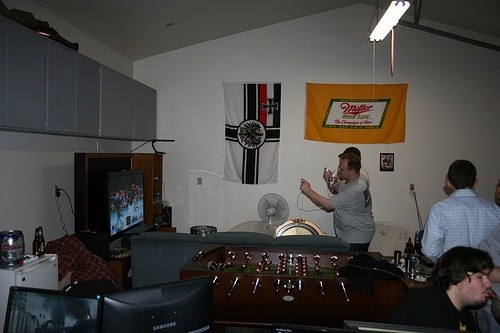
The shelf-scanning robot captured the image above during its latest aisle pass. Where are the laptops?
[3,285,105,333]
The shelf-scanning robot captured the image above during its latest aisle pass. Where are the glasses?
[339,167,354,172]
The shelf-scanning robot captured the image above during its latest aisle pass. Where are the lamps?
[370,0,410,42]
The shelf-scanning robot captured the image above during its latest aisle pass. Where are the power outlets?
[55,186,58,195]
[409,184,415,190]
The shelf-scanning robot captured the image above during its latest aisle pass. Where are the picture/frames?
[380,153,394,172]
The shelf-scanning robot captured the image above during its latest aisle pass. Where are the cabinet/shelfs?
[74,151,176,284]
[0,16,156,142]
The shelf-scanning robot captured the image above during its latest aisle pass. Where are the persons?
[386,246,495,330]
[421,159,500,263]
[113,183,143,225]
[301,147,376,252]
[477,178,500,333]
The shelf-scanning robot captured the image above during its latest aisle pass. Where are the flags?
[305,82,408,144]
[222,81,281,184]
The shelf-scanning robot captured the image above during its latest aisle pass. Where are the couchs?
[128,230,351,288]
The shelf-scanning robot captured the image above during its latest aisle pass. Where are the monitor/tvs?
[101,275,214,333]
[100,168,146,240]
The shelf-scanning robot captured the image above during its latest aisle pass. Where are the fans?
[257,193,289,228]
